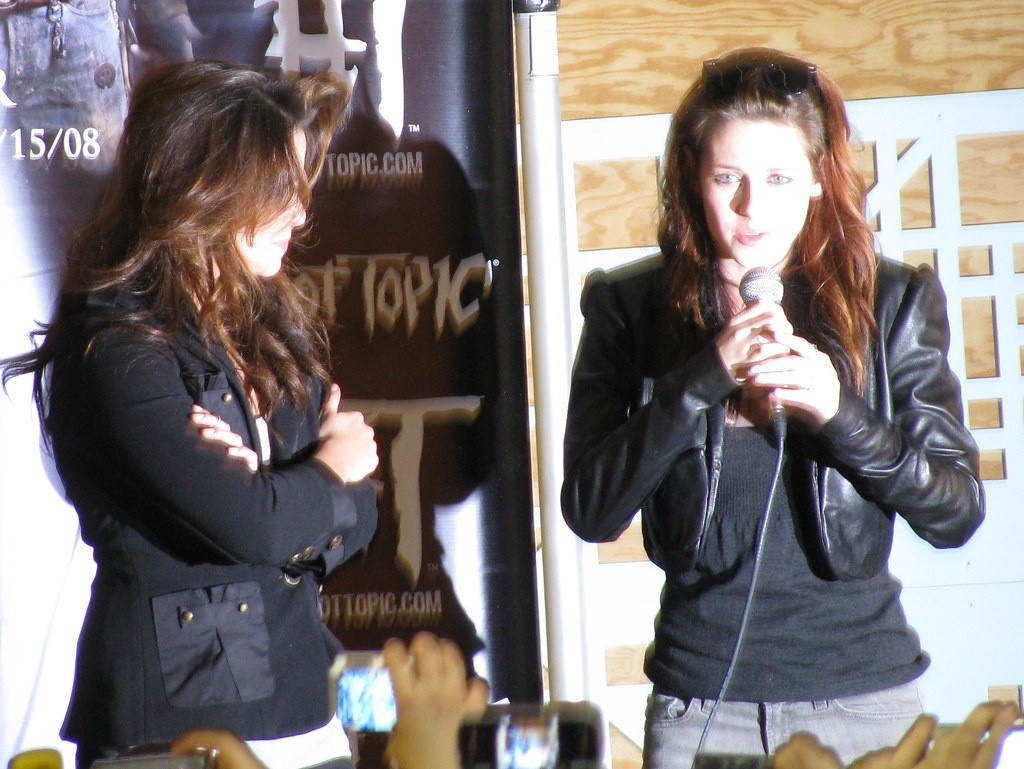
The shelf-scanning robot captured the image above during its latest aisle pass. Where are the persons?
[560,46,987,769]
[0,57,381,769]
[174,632,1020,769]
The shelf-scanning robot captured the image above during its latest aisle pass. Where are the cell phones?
[327,653,399,732]
[451,701,612,769]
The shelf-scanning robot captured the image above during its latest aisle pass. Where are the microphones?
[740,266,786,440]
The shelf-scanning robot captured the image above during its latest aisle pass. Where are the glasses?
[701,59,822,99]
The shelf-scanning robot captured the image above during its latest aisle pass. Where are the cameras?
[91,752,214,769]
